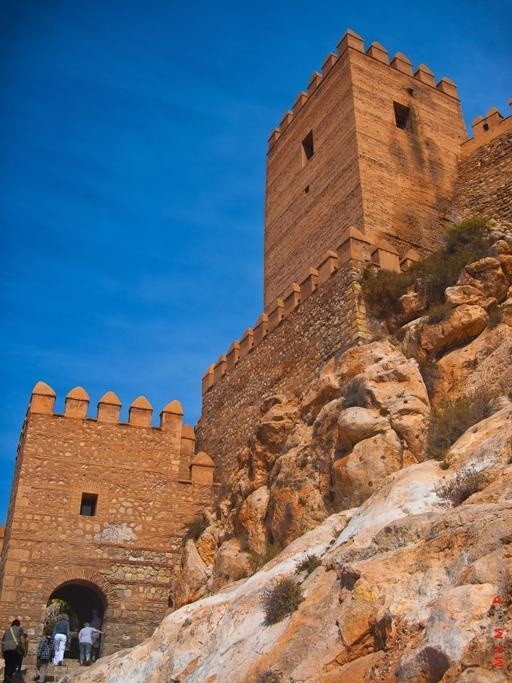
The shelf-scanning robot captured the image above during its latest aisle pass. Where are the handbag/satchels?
[15,642,24,655]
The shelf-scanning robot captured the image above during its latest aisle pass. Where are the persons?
[89,611,101,662]
[1,619,27,682]
[37,627,55,683]
[52,614,70,666]
[78,623,104,666]
